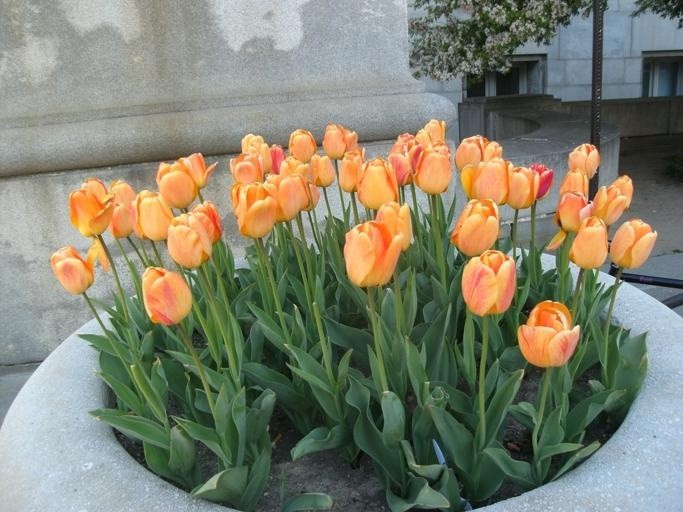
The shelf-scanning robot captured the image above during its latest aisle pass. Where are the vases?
[1,248,683,511]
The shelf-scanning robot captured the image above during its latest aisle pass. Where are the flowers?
[52,118,657,512]
